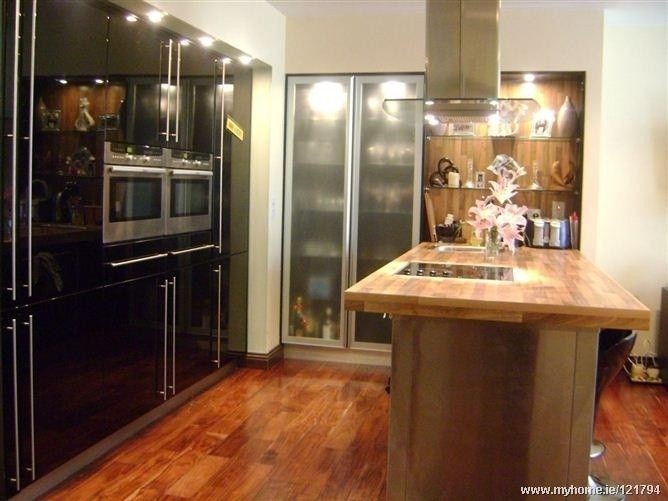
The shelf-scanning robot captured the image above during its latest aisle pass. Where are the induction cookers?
[400,261,515,282]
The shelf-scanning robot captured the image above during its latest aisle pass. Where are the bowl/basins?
[436,224,459,241]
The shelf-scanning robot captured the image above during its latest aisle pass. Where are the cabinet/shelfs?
[213,250,251,376]
[0,287,113,500]
[110,6,216,152]
[416,73,585,249]
[214,58,254,261]
[0,0,114,311]
[105,263,214,440]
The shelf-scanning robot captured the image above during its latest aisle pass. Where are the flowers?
[459,148,529,253]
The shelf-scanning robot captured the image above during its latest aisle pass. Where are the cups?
[448,172,459,187]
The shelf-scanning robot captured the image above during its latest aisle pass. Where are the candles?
[447,171,459,189]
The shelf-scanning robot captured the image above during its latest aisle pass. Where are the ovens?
[102,139,214,255]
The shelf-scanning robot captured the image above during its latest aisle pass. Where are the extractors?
[382,0,540,130]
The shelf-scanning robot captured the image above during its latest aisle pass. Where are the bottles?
[36,99,50,130]
[289,297,339,339]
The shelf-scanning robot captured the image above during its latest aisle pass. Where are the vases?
[483,231,504,261]
